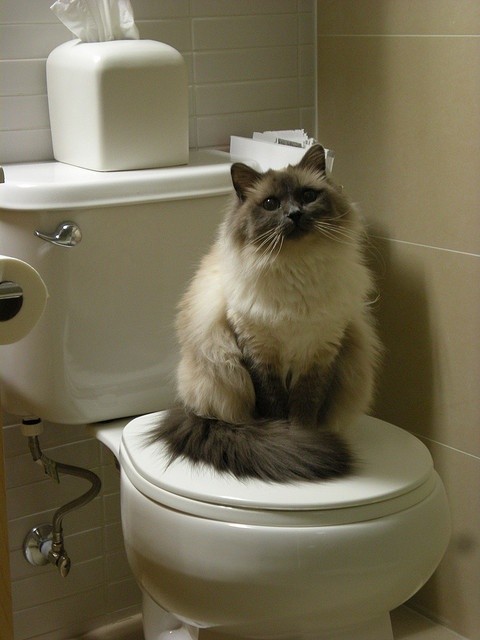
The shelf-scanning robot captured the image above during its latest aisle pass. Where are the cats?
[136,142,385,487]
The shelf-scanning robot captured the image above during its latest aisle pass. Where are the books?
[228,127,335,180]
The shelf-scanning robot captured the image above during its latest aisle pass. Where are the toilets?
[0,149,452,640]
